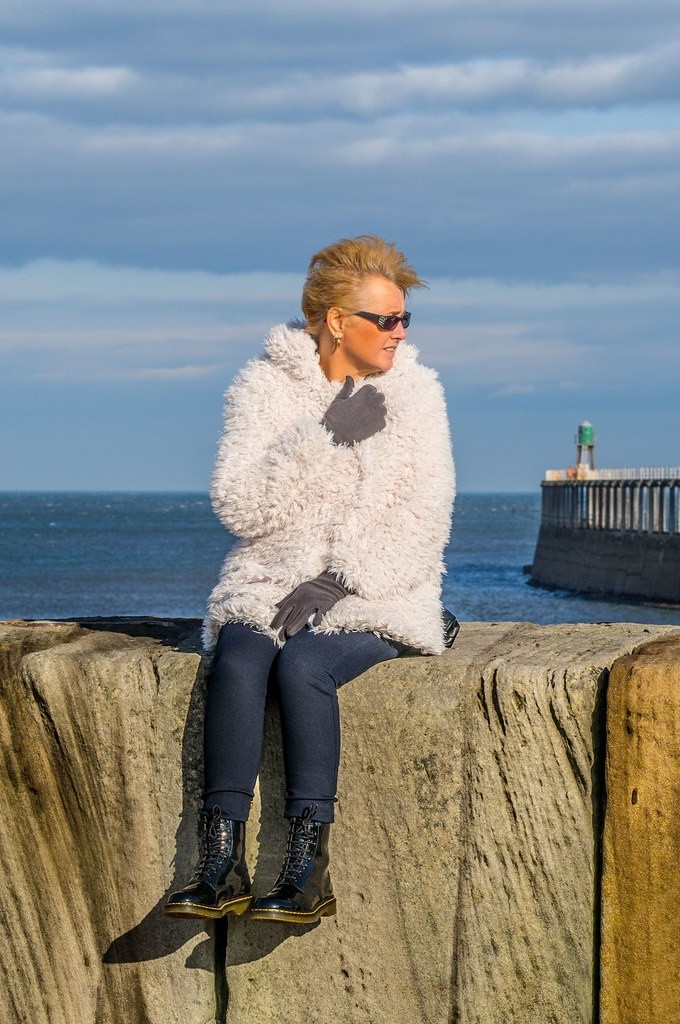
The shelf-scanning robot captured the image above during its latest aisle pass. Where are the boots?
[164,803,337,923]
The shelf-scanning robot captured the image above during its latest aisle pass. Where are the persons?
[162,235,456,926]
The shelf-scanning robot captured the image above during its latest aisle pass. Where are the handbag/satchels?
[441,608,460,648]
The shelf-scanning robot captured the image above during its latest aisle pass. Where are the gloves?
[270,566,356,637]
[319,375,387,448]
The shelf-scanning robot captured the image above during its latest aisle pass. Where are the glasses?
[324,307,411,330]
